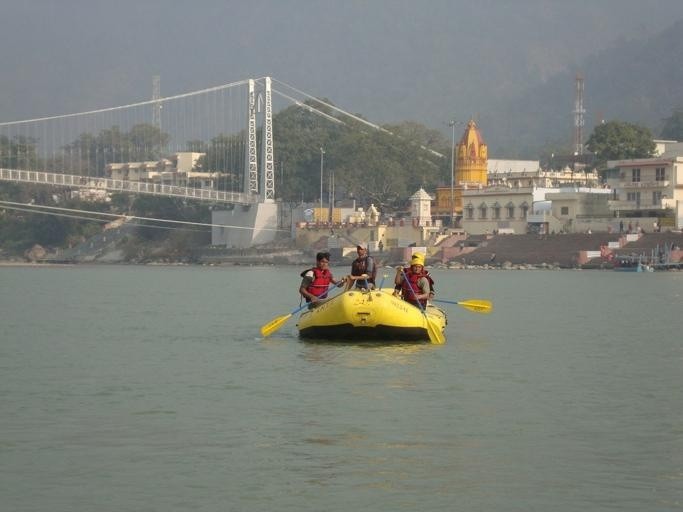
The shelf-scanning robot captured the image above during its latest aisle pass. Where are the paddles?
[401,266,446,345]
[260,278,348,337]
[432,298,491,314]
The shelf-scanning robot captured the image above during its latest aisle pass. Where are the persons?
[299,252,344,309]
[392,252,435,310]
[348,242,377,290]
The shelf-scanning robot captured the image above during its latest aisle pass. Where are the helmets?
[409,252,425,267]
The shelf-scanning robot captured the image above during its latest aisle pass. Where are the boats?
[613,254,648,272]
[296,288,448,342]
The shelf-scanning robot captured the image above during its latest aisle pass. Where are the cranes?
[550,151,598,184]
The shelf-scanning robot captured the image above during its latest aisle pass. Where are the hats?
[355,241,367,251]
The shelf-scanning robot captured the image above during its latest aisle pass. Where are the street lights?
[319,147,326,224]
[448,119,458,228]
